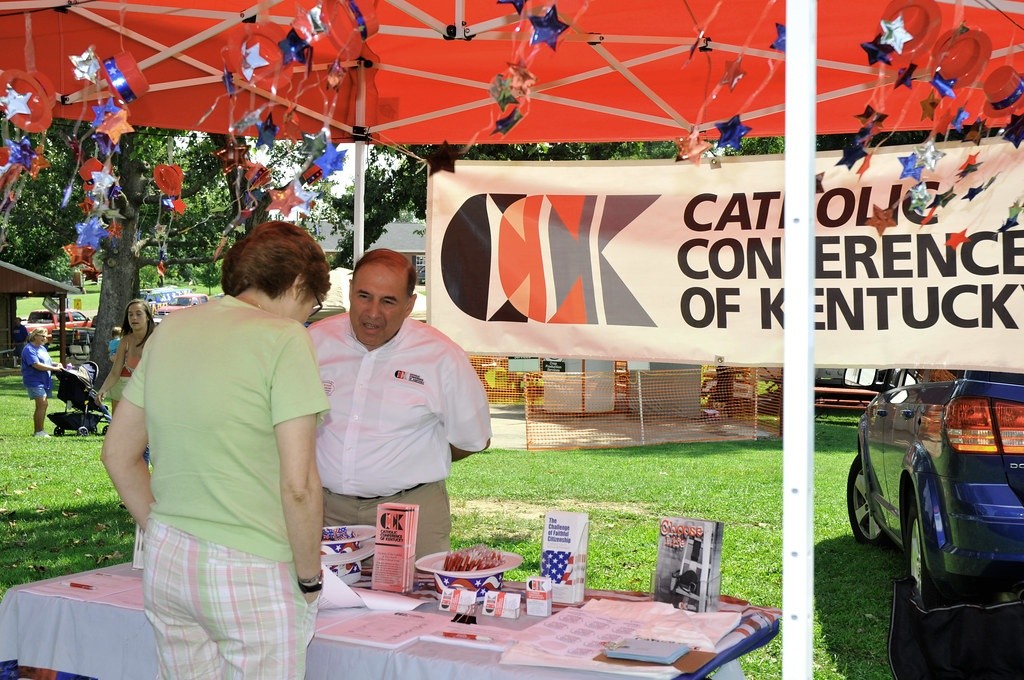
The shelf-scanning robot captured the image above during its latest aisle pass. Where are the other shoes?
[34,431,50,437]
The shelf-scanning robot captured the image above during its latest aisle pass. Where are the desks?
[0,561,782,680]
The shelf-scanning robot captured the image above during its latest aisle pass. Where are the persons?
[100,221,330,680]
[12,318,29,368]
[95,298,156,506]
[21,327,63,437]
[305,248,493,564]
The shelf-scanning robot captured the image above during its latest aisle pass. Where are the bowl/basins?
[414,552,524,602]
[321,525,376,586]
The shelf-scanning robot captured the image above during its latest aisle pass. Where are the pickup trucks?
[50,315,99,356]
[20,310,94,348]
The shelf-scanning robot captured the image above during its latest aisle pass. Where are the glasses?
[310,290,323,316]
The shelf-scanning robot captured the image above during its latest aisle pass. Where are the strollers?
[51,359,115,436]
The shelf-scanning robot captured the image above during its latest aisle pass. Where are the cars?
[156,294,209,318]
[303,304,346,328]
[846,371,1024,601]
[141,287,192,306]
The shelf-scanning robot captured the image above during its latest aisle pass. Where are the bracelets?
[298,570,324,594]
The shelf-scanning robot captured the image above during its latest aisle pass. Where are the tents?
[0,0,1024,680]
